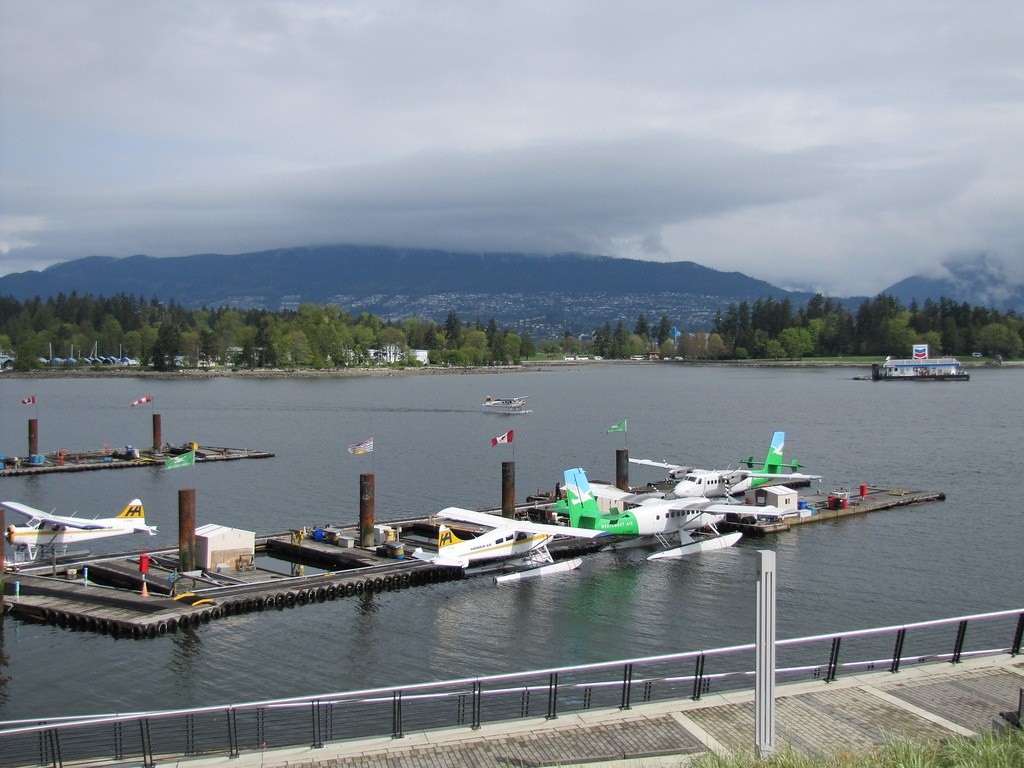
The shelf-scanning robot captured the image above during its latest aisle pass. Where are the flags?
[348,437,374,454]
[23,396,37,405]
[166,451,195,469]
[492,430,513,447]
[131,396,151,407]
[607,420,625,433]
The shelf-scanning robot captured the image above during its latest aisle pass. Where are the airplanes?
[411,506,613,586]
[629,429,823,500]
[482,395,532,414]
[0,497,159,567]
[545,467,780,562]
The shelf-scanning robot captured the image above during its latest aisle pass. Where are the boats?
[882,355,970,381]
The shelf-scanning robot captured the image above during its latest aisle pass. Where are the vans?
[972,353,982,358]
